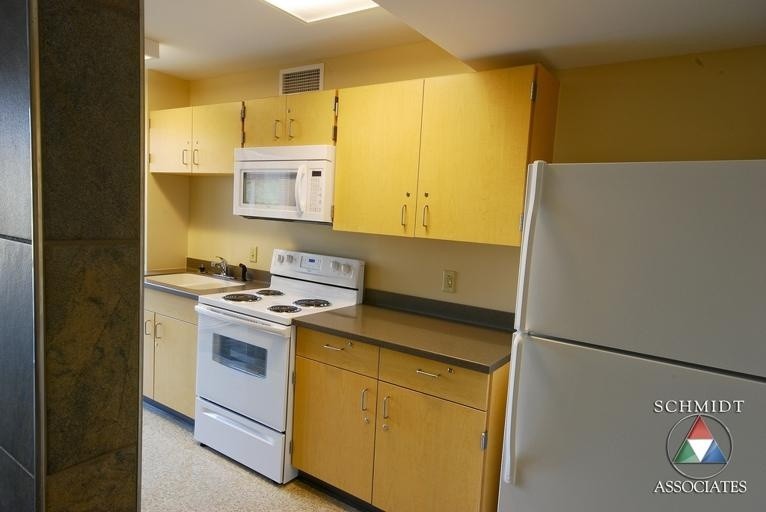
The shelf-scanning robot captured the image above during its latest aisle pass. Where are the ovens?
[194,302,298,485]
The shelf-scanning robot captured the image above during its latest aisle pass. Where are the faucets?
[210,256,228,277]
[238,263,248,281]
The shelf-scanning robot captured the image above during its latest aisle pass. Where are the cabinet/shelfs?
[242,89,333,148]
[142,284,198,419]
[333,62,560,249]
[291,327,510,512]
[147,102,242,175]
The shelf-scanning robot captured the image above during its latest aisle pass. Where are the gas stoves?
[199,276,361,327]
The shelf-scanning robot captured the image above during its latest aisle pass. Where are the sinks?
[146,272,217,286]
[184,280,246,291]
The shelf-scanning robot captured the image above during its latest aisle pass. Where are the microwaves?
[232,144,335,223]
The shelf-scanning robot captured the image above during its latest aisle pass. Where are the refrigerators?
[496,159,765,510]
